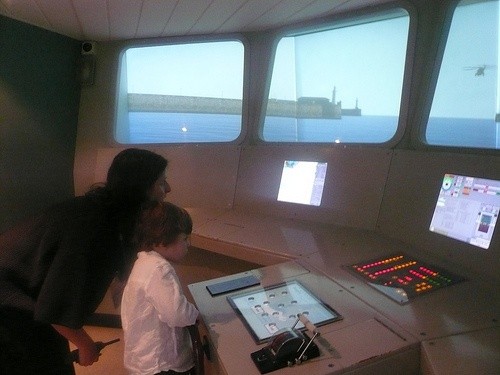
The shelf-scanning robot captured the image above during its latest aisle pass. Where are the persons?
[120,201,207,375]
[0,147,170,375]
